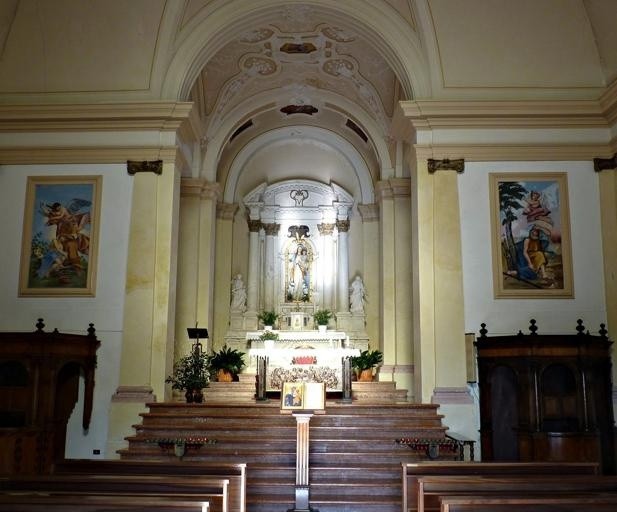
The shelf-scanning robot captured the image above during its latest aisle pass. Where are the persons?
[296,248,308,274]
[36,199,92,285]
[288,244,309,302]
[231,273,247,312]
[521,191,552,223]
[507,225,549,280]
[349,276,366,312]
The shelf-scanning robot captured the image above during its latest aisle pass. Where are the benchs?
[401,460,615,511]
[0,457,248,512]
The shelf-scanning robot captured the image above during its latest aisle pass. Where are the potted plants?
[258,311,277,330]
[210,347,243,381]
[165,355,210,404]
[261,333,276,348]
[314,310,329,330]
[349,351,382,381]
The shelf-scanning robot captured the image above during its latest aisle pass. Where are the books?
[282,382,324,410]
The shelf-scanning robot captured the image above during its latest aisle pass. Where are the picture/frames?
[17,174,102,298]
[487,171,573,299]
